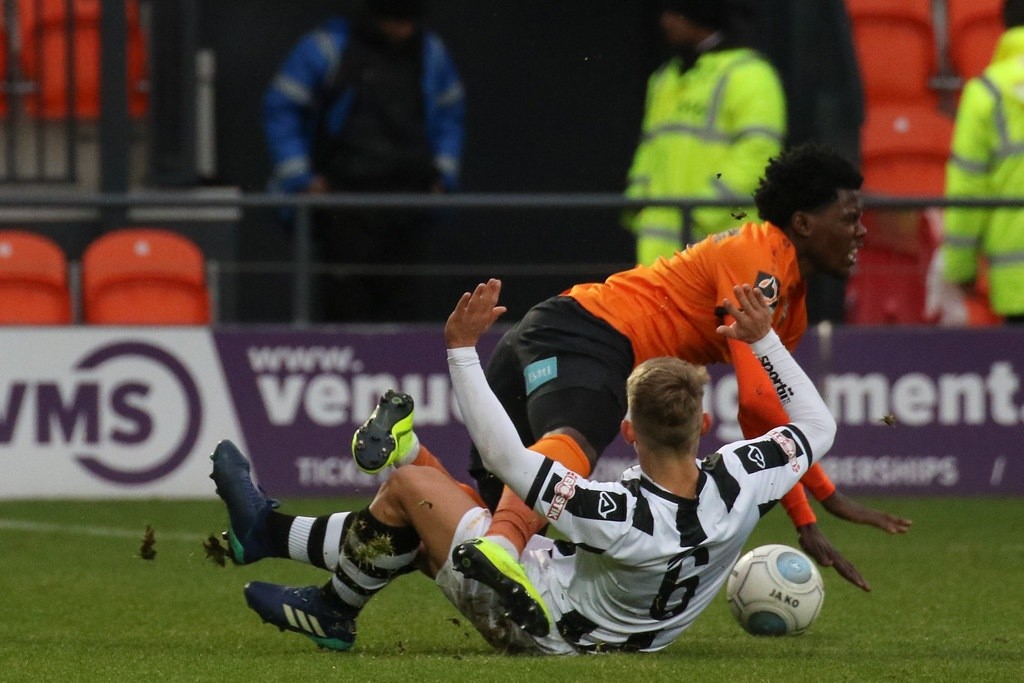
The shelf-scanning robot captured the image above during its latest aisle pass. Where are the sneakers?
[351,388,415,474]
[208,440,280,566]
[452,536,550,638]
[244,580,357,652]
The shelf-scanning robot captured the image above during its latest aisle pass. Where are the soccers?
[726,544,824,636]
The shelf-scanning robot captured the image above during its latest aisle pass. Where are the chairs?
[860,102,954,239]
[81,228,221,326]
[843,0,940,106]
[0,227,83,325]
[940,0,1008,110]
[13,0,152,123]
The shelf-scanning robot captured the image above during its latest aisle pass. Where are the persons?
[209,278,837,655]
[257,0,466,324]
[620,0,788,270]
[943,0,1024,327]
[351,143,912,638]
